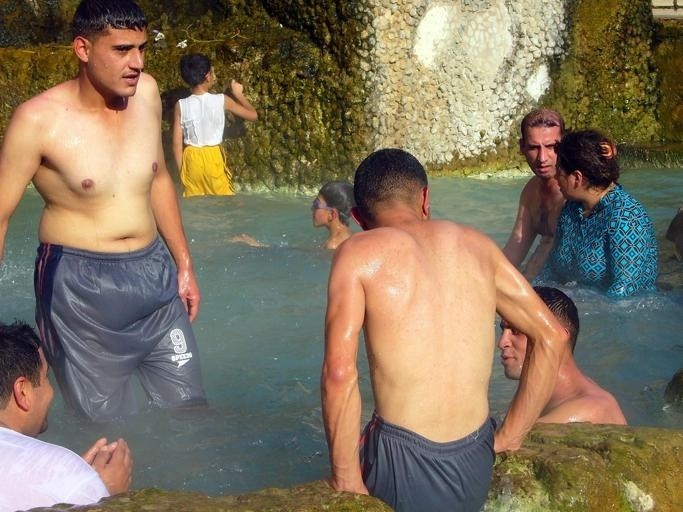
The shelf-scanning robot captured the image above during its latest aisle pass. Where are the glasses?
[313,198,347,218]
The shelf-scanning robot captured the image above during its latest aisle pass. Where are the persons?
[537,129,658,299]
[500,109,569,280]
[320,148,566,512]
[1,0,207,422]
[498,285,629,426]
[0,321,132,512]
[228,180,355,249]
[172,53,258,198]
